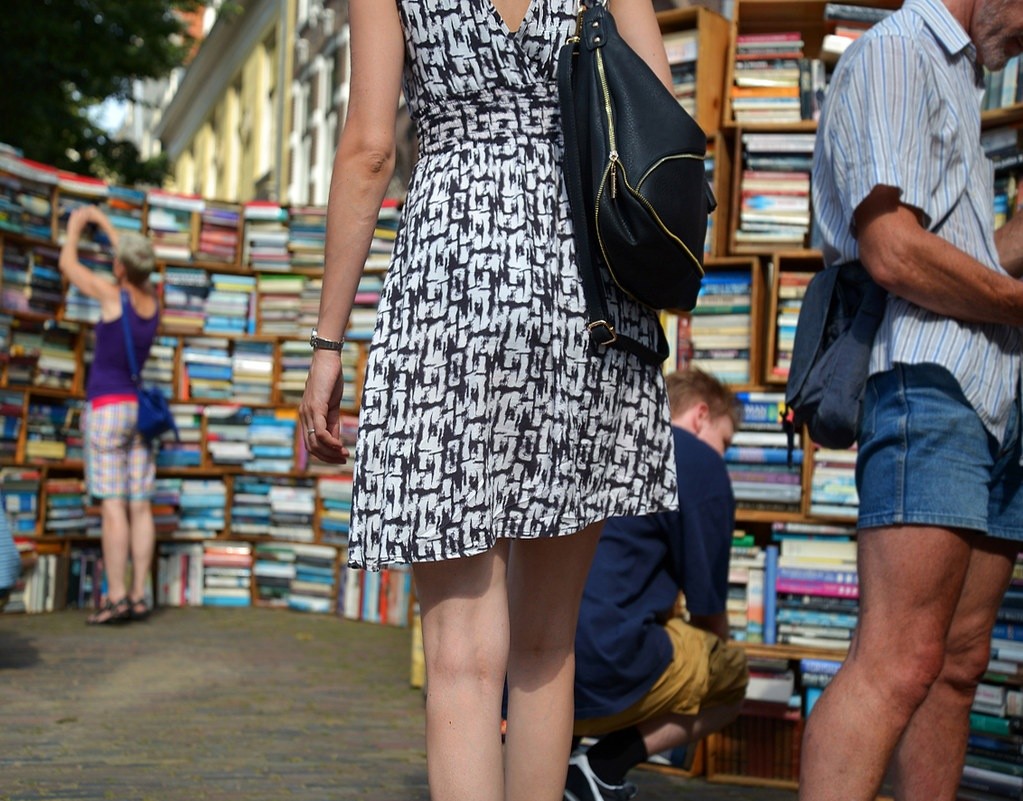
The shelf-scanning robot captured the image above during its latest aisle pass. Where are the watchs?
[310,327,345,350]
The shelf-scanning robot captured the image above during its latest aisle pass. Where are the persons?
[499,369,751,801]
[797,0,1022,801]
[298,1,676,801]
[59,207,161,624]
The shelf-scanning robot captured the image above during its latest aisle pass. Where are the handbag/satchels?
[786,258,887,451]
[138,385,182,447]
[557,1,719,366]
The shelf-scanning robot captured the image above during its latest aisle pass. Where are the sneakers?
[562,754,637,801]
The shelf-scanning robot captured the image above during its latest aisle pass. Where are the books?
[0,0,1023,801]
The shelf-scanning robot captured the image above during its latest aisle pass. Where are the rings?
[308,429,315,435]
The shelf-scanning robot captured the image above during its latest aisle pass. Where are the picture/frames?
[704,707,807,791]
[634,706,703,776]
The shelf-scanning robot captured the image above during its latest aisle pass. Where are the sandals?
[86,597,150,625]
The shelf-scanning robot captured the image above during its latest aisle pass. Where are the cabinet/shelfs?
[650,0,1023,798]
[0,163,410,628]
